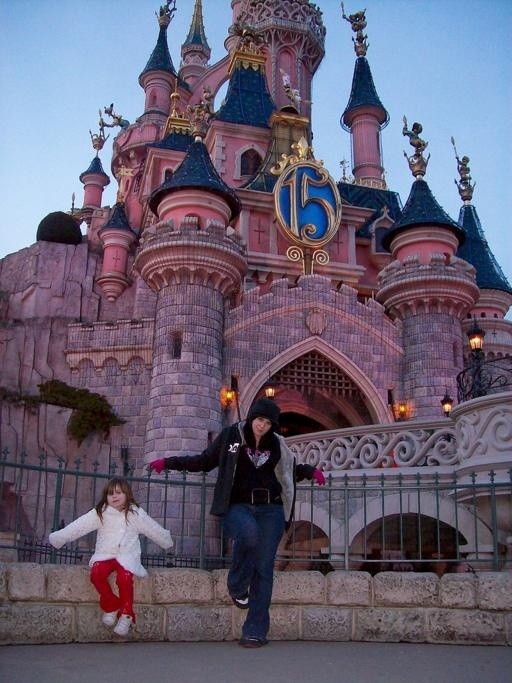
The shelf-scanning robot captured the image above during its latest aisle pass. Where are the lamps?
[398,402,407,421]
[224,388,234,412]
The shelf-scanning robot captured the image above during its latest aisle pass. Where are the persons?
[150,397,325,647]
[48,476,175,635]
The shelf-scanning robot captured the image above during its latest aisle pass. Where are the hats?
[246,397,280,425]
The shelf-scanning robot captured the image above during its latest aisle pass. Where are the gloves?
[149,457,166,474]
[312,469,326,486]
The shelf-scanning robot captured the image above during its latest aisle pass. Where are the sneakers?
[102,609,119,627]
[232,590,249,609]
[113,613,133,637]
[241,637,269,649]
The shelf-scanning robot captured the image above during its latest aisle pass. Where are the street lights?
[440,388,453,442]
[466,319,485,394]
[263,379,277,400]
